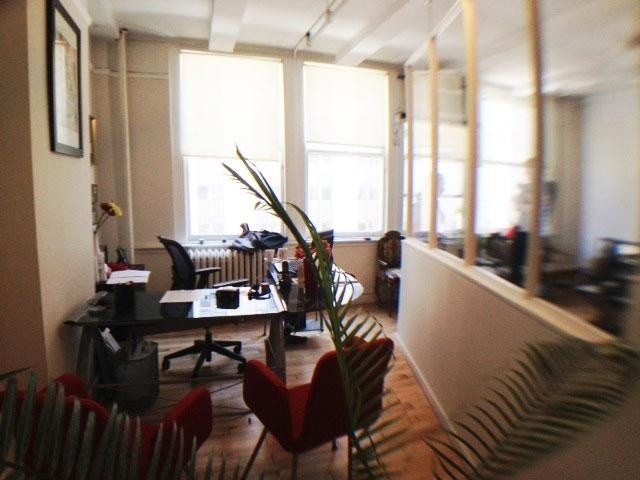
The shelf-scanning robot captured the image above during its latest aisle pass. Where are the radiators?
[185,248,268,290]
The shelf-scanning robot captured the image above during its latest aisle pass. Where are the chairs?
[374,231,406,316]
[155,235,249,381]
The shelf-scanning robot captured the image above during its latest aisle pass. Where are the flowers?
[91,201,119,232]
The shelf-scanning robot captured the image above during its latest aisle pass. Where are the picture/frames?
[46,0,85,157]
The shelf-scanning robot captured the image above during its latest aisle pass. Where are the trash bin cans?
[108,340,159,415]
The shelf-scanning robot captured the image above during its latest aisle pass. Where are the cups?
[278,248,287,261]
[264,249,275,261]
